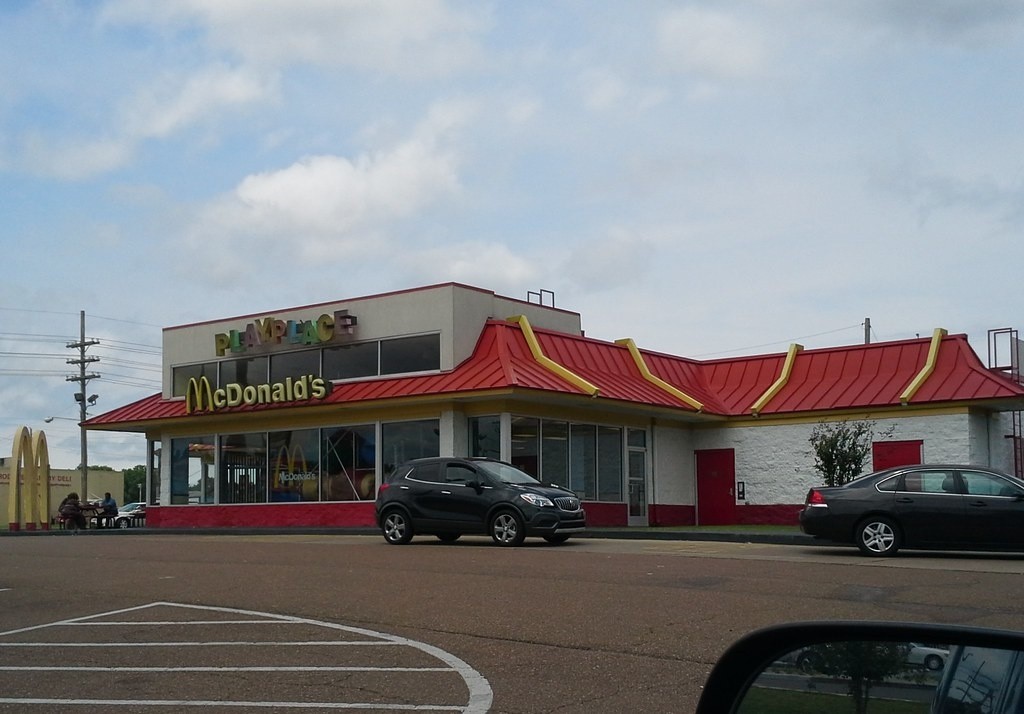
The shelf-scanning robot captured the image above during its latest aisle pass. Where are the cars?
[90,501,147,527]
[773,647,828,673]
[798,462,1024,565]
[694,619,1024,714]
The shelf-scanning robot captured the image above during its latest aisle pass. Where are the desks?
[79,506,103,528]
[129,512,146,527]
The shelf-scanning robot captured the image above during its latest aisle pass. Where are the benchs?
[90,515,118,528]
[127,516,145,527]
[56,516,81,530]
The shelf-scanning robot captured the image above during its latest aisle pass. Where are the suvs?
[373,456,588,548]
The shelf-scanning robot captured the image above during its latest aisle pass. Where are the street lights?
[44,415,87,504]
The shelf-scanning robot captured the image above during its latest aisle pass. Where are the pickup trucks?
[875,640,951,670]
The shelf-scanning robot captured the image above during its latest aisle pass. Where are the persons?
[95,492,118,530]
[57,494,69,514]
[61,492,88,530]
